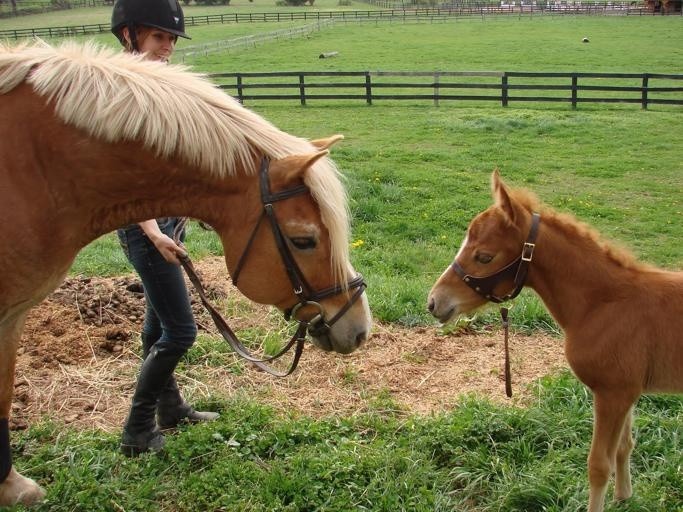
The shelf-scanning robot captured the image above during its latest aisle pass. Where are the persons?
[109,0,220,457]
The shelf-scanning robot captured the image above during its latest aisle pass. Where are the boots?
[117,330,221,455]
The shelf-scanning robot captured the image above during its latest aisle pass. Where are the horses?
[425,165,683,512]
[0,32,377,508]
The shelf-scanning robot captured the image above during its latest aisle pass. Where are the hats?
[110,0,193,40]
